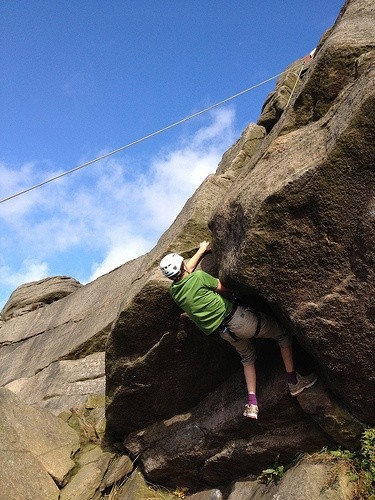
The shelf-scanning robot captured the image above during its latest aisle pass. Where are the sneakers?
[288,370,319,397]
[242,403,259,419]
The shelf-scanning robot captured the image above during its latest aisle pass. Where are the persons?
[159,240,318,419]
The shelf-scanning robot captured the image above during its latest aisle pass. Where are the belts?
[220,303,237,325]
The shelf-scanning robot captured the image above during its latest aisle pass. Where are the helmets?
[159,252,184,278]
